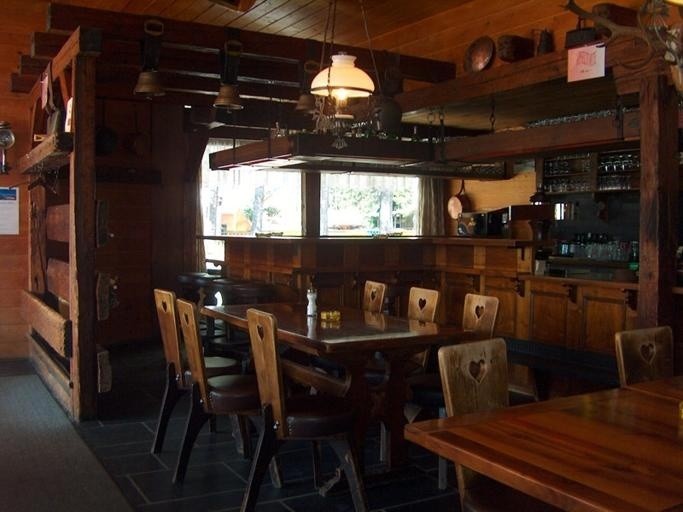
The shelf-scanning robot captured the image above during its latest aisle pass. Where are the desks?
[402,374,682,512]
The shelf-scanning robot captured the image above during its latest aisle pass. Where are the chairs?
[438,337,553,511]
[240,308,368,511]
[615,326,674,386]
[153,279,539,490]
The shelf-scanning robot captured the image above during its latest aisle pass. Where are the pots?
[446,180,470,220]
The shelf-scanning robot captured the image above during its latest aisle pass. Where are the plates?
[460,35,495,74]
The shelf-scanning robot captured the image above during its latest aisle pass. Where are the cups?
[541,149,639,195]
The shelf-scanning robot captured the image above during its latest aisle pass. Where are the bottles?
[305,288,318,316]
[533,247,545,276]
[557,231,640,272]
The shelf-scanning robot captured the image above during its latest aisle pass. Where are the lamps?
[307,48,375,104]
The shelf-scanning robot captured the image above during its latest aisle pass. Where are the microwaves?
[456,203,542,239]
